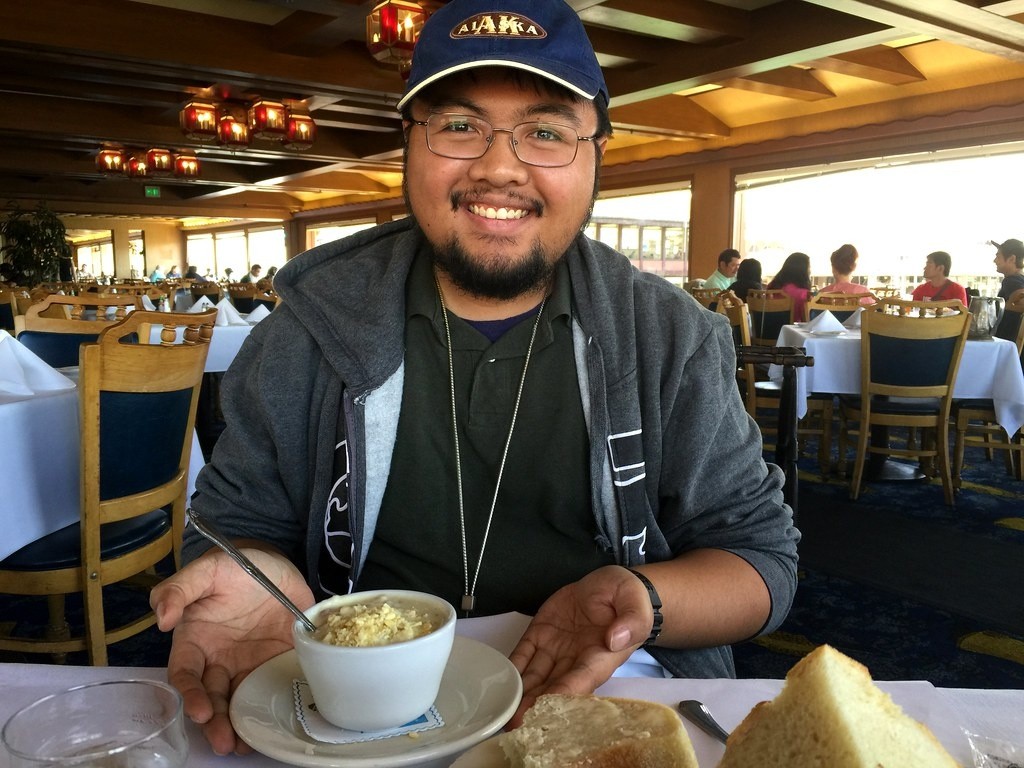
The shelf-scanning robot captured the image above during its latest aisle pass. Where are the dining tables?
[0,665,1024,768]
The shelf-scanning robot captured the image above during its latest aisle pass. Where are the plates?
[450,731,506,767]
[229,636,525,768]
[794,321,854,337]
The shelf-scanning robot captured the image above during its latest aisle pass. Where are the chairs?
[0,278,279,666]
[684,276,1024,500]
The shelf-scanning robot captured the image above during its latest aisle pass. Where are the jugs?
[966,295,1006,342]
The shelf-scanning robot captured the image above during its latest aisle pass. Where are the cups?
[292,588,459,733]
[0,678,191,768]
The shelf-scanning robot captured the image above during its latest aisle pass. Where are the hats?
[991,239,1024,268]
[398,1,611,108]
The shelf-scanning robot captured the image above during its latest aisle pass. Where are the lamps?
[95,0,429,179]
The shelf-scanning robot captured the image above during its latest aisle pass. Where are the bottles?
[157,293,171,312]
[202,302,210,312]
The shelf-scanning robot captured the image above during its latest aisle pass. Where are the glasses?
[405,112,605,168]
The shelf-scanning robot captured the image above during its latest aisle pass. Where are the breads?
[717,645,963,768]
[497,693,701,768]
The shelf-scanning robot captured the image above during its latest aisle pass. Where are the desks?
[784,325,1016,401]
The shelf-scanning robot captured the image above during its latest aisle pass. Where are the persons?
[818,244,880,306]
[150,264,166,282]
[616,564,663,652]
[911,251,968,308]
[702,249,741,299]
[256,266,278,290]
[766,253,816,325]
[167,266,183,278]
[221,268,236,282]
[77,264,95,278]
[149,0,802,757]
[725,259,762,305]
[184,266,202,278]
[203,269,214,281]
[240,264,261,284]
[941,239,1024,409]
